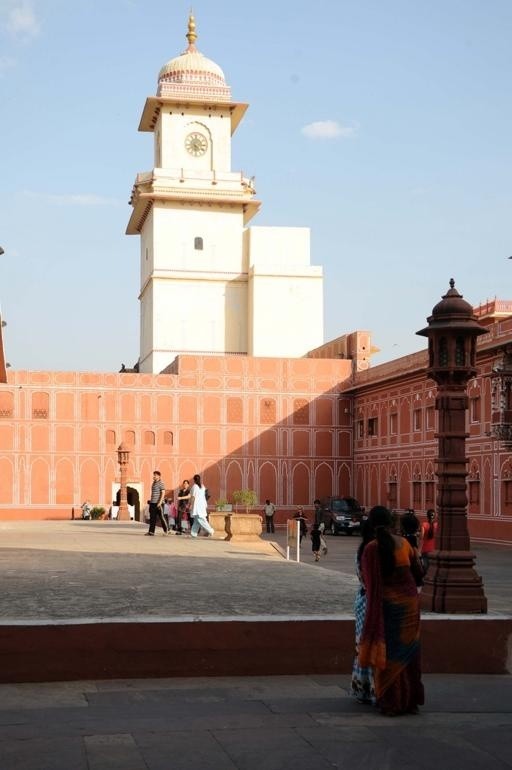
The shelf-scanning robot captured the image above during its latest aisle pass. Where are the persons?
[313,499,327,556]
[186,474,214,538]
[261,499,277,533]
[144,471,168,536]
[176,479,200,535]
[352,504,438,714]
[293,505,309,547]
[144,497,177,531]
[310,524,327,561]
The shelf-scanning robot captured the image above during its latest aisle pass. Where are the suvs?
[320,494,367,537]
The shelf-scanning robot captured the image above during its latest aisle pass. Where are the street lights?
[414,278,489,613]
[114,439,133,520]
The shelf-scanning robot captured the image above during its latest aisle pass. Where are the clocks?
[185,132,208,157]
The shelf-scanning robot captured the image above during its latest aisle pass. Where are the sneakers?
[145,532,154,536]
[208,528,214,537]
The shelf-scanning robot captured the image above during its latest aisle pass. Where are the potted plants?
[90,507,107,520]
[207,488,264,546]
